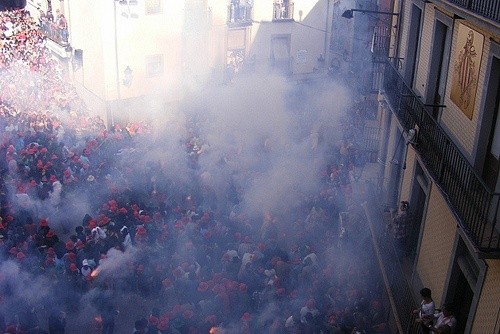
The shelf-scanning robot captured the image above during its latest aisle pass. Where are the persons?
[393,200,410,270]
[422,301,457,334]
[412,287,435,328]
[1,0,390,334]
